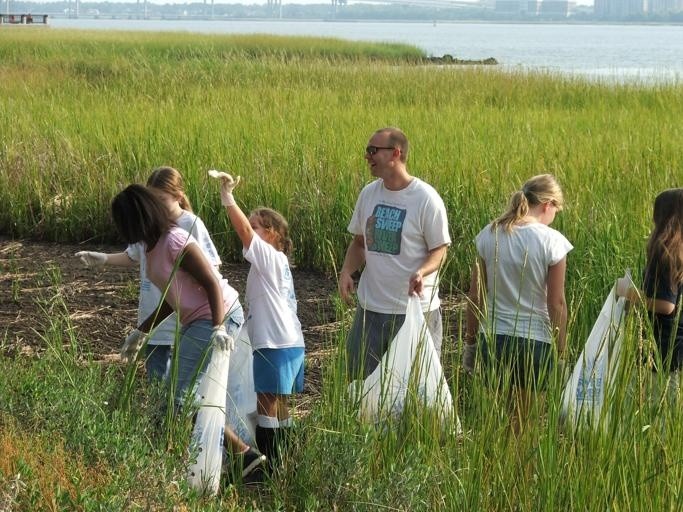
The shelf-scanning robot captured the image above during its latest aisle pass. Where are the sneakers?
[225,445,266,489]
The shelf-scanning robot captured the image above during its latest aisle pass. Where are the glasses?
[365,145,403,155]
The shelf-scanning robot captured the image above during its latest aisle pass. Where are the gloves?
[615,267,633,298]
[74,251,108,268]
[461,342,479,377]
[216,172,240,207]
[120,328,150,365]
[210,324,234,352]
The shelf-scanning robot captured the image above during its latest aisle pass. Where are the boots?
[255,423,282,480]
[278,425,298,461]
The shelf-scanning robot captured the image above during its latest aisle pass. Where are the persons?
[73,165,223,386]
[459,171,574,441]
[614,188,682,395]
[206,169,307,477]
[333,125,453,388]
[108,182,268,484]
